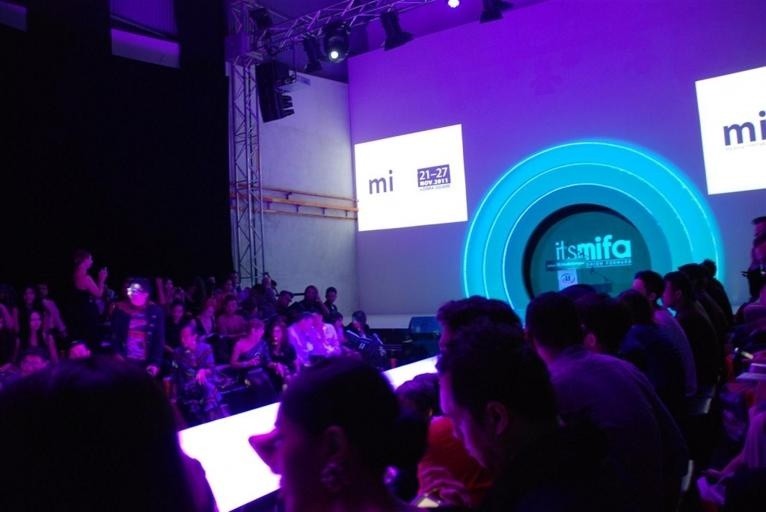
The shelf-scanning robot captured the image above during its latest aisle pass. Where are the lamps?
[380,8,413,51]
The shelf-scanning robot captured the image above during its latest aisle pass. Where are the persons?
[391,261,765,510]
[2,248,397,430]
[746,220,765,293]
[0,355,223,509]
[272,351,422,512]
